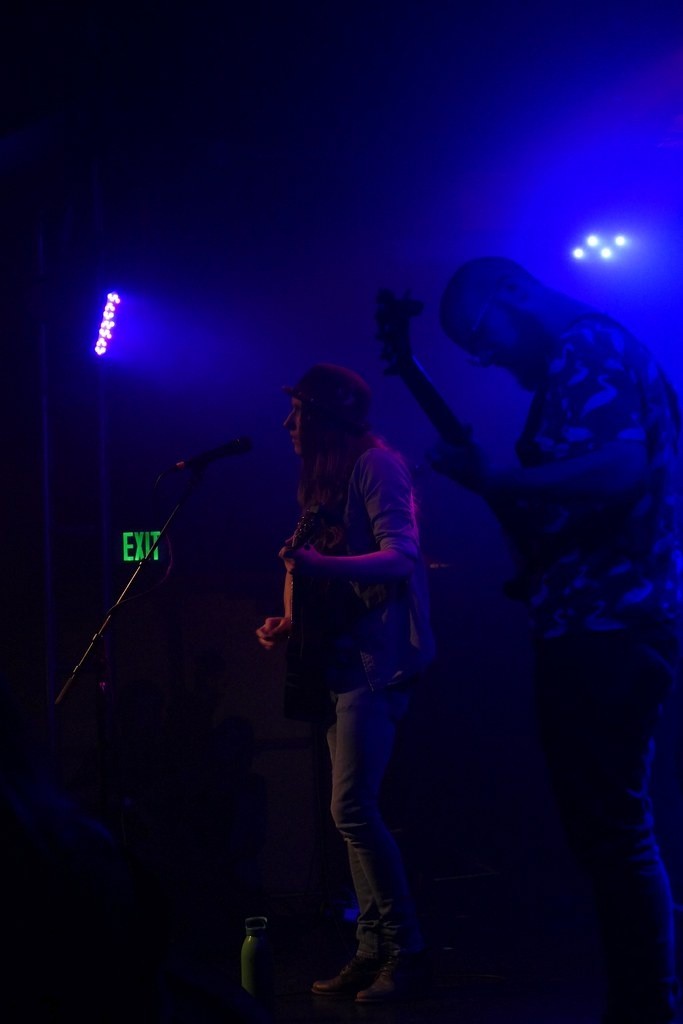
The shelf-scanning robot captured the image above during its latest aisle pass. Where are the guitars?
[270,497,338,722]
[372,286,580,603]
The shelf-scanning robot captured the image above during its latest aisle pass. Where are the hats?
[283,364,373,432]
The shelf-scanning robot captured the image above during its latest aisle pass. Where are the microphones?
[168,436,252,471]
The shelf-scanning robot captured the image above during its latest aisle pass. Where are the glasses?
[461,286,498,358]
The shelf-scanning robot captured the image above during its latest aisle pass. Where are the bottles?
[241,917,268,995]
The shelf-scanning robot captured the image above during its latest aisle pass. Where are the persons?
[426,256,683,1024]
[254,363,437,1004]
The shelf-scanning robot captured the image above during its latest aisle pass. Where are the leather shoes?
[355,961,431,1001]
[311,960,377,994]
